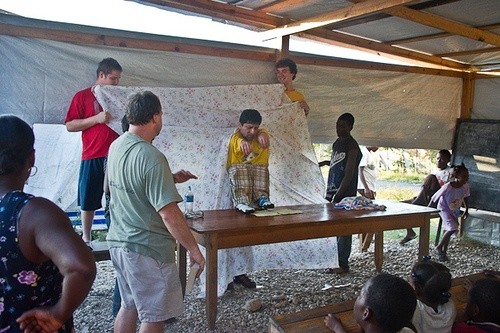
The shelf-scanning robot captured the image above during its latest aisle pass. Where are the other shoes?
[236,203,255,213]
[323,267,347,274]
[233,275,256,287]
[257,196,274,209]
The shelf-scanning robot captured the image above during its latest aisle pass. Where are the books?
[185,263,200,293]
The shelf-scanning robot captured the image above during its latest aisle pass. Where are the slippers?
[398,235,419,244]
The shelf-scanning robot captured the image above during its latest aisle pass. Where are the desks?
[177,200,500,333]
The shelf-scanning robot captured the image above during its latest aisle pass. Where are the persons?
[323,273,418,333]
[0,115,97,332]
[454,276,500,333]
[65,57,122,248]
[226,109,275,213]
[399,149,455,245]
[411,259,456,332]
[324,113,363,274]
[104,113,199,319]
[357,146,380,200]
[277,58,309,116]
[427,165,470,262]
[103,90,205,333]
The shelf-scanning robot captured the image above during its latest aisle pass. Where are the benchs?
[92,196,464,262]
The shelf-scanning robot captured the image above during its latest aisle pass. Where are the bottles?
[184,185,194,214]
